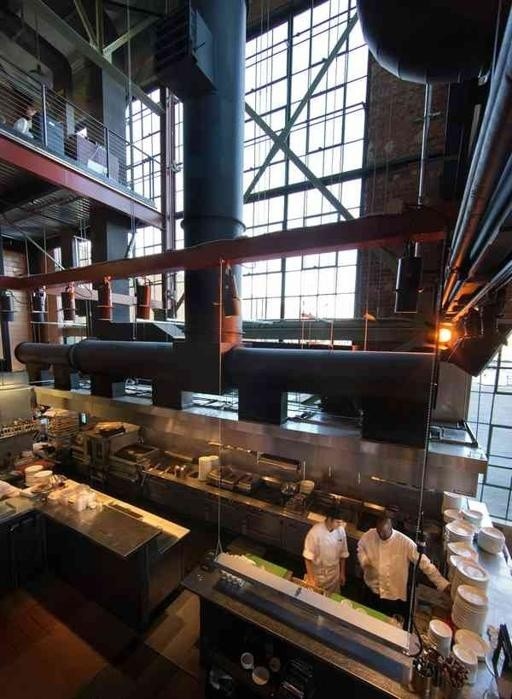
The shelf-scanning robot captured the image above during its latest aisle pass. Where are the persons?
[24,102,45,141]
[357,517,452,617]
[302,505,351,594]
[0,478,22,502]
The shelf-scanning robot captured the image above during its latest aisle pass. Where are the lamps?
[433,303,483,353]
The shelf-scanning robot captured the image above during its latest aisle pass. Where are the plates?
[298,478,315,494]
[429,488,508,684]
[24,465,60,491]
[197,453,221,486]
